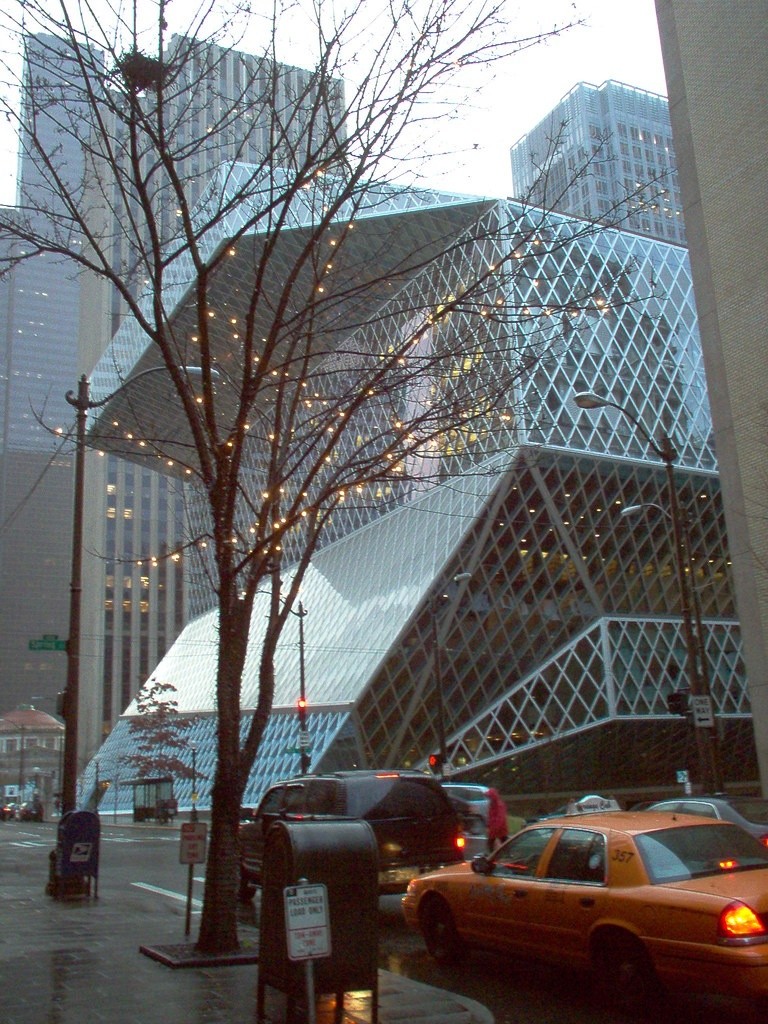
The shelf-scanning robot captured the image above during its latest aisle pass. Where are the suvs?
[235,771,466,909]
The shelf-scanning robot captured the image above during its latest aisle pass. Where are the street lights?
[238,588,308,775]
[429,755,439,767]
[573,389,717,796]
[429,570,474,763]
[0,718,23,805]
[621,502,718,794]
[56,365,222,812]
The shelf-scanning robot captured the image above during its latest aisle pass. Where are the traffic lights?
[298,699,307,722]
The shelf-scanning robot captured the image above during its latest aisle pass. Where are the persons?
[484,788,509,853]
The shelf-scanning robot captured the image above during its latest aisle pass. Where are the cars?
[522,793,668,829]
[14,802,44,822]
[399,809,768,1024]
[440,781,492,835]
[0,802,18,821]
[645,798,768,848]
